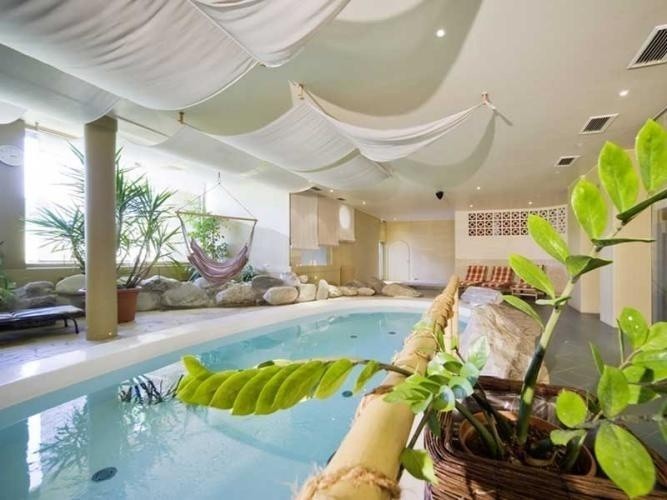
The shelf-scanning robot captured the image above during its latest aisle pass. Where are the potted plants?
[17,139,184,324]
[172,118,667,500]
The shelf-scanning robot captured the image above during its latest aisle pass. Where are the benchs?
[0,303,85,333]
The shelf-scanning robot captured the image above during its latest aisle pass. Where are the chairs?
[510,264,546,303]
[457,265,487,298]
[482,265,514,298]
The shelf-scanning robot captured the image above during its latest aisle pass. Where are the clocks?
[0,145,24,168]
